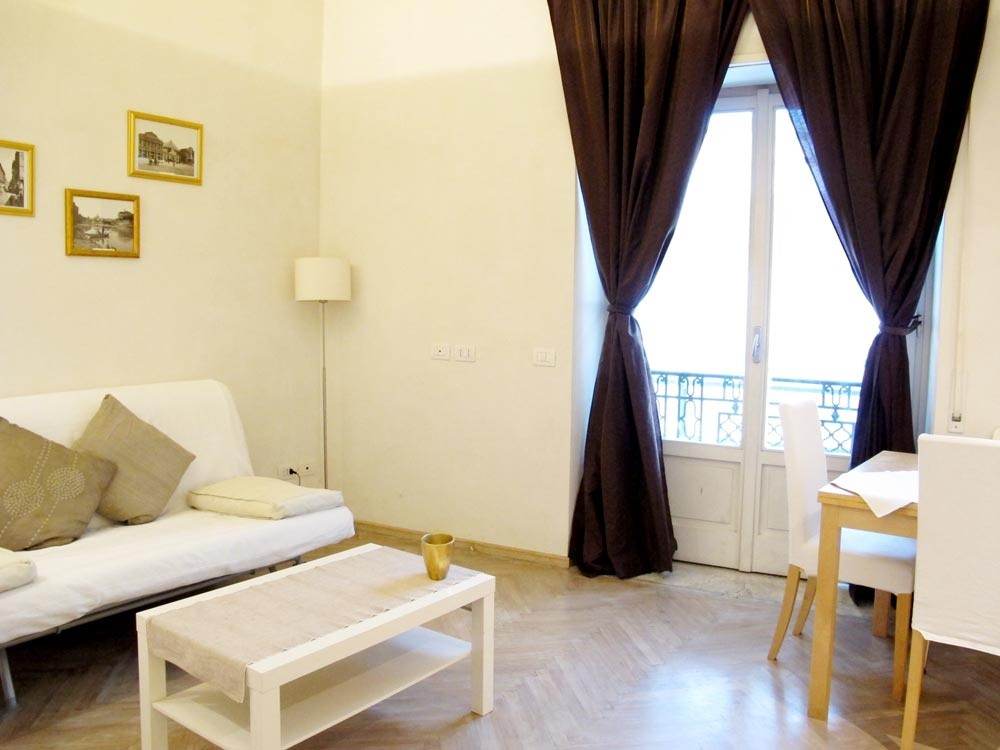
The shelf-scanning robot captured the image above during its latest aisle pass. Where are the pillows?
[69,393,196,525]
[188,477,343,520]
[0,417,117,551]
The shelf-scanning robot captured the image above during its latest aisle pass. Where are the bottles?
[946,413,963,436]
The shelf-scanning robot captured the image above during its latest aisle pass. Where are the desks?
[807,451,918,720]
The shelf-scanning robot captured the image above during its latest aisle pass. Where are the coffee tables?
[135,542,496,750]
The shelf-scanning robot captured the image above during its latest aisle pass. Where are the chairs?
[899,432,1000,750]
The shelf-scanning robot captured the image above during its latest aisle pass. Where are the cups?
[421,533,456,580]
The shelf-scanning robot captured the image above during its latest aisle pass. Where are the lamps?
[296,257,351,489]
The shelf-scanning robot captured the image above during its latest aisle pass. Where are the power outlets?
[278,464,296,479]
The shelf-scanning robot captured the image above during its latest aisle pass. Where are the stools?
[768,528,918,703]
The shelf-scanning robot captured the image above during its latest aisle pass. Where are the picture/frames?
[0,140,35,216]
[65,188,140,258]
[127,110,203,185]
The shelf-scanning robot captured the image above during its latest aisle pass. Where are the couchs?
[0,380,356,708]
